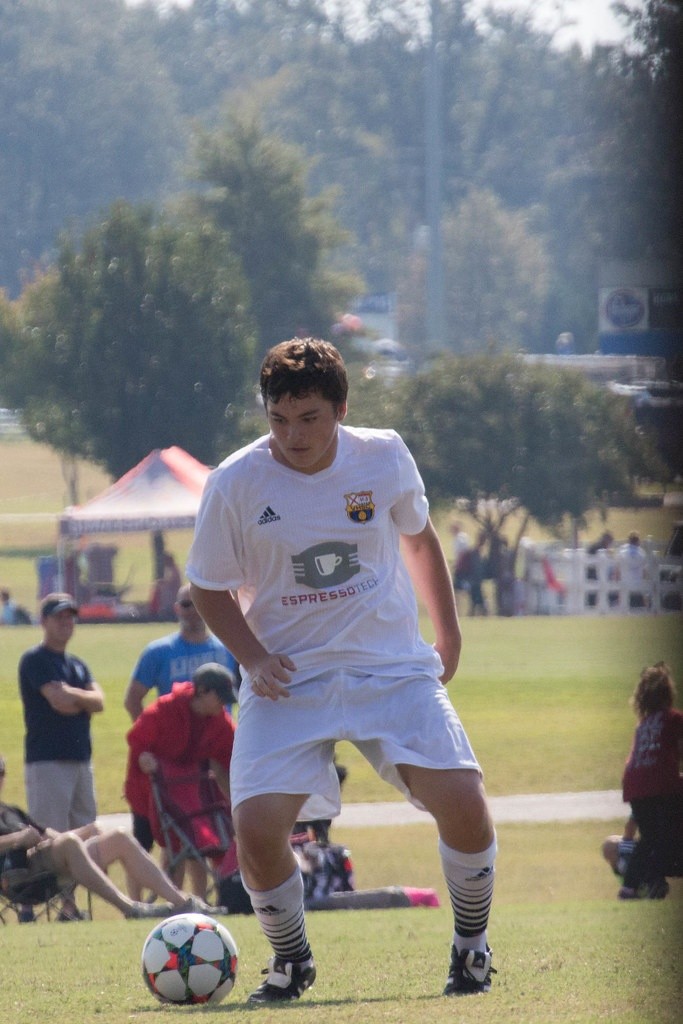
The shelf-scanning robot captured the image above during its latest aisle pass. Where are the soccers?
[142,913,239,1004]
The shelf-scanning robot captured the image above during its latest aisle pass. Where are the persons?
[123,582,243,725]
[585,529,614,606]
[63,548,82,599]
[619,530,648,607]
[16,592,103,923]
[0,757,229,923]
[459,531,489,616]
[519,535,539,616]
[0,589,33,625]
[618,661,683,898]
[602,810,641,877]
[185,334,498,1004]
[120,662,238,921]
[448,521,471,606]
[161,551,182,622]
[494,534,517,617]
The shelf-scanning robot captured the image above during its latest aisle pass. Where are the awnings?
[58,445,213,538]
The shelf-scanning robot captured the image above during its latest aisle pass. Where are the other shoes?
[648,881,669,899]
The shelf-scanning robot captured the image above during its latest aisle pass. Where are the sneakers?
[124,901,173,917]
[171,895,228,915]
[247,954,316,1003]
[441,941,498,997]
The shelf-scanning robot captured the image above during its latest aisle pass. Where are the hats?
[42,598,79,617]
[193,662,238,704]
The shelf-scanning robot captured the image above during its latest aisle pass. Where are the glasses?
[178,599,195,607]
